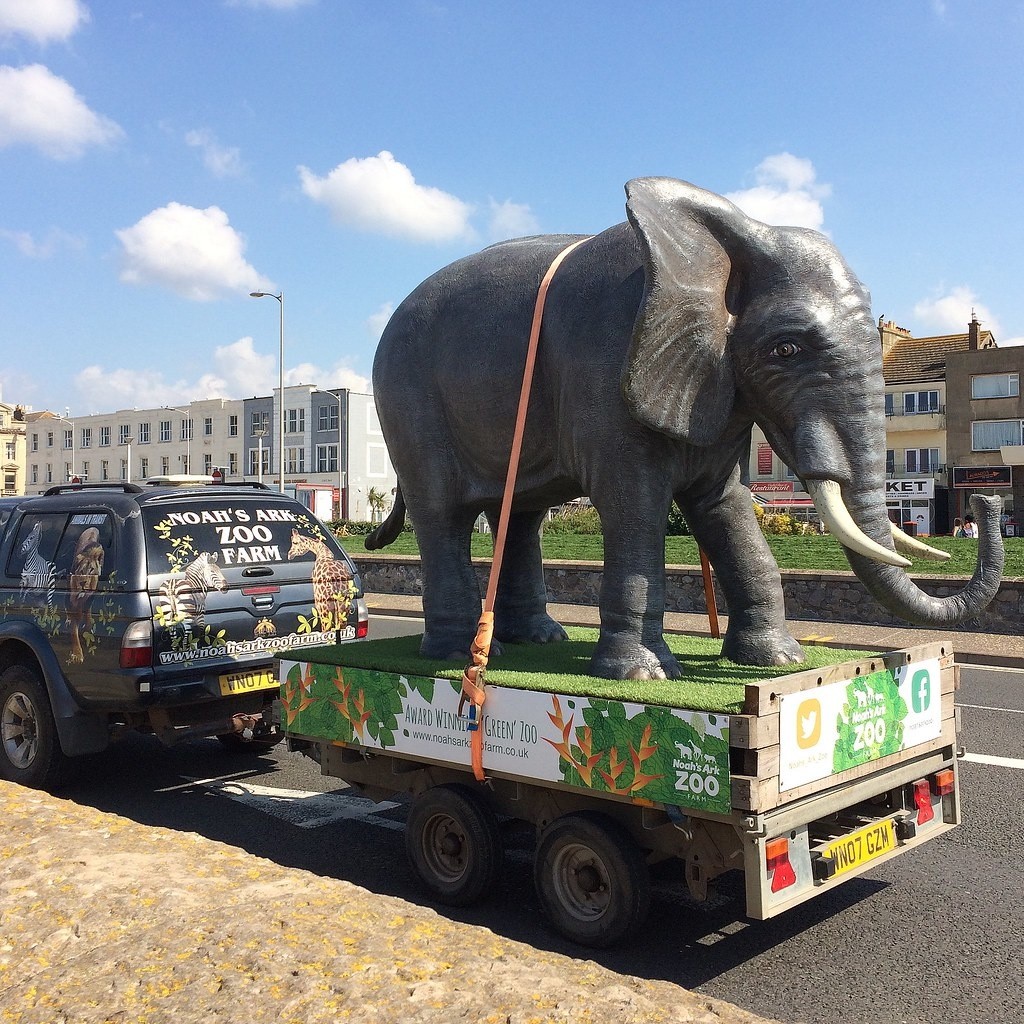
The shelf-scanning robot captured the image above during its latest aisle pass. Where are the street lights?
[165,406,190,475]
[124,436,136,484]
[50,416,74,481]
[314,389,343,520]
[249,288,286,493]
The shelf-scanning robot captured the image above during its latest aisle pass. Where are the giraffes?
[287,528,353,632]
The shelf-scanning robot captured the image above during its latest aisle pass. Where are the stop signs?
[212,471,222,485]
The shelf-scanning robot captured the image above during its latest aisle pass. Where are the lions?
[65,527,104,664]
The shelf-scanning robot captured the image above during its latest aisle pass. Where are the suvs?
[0,472,368,789]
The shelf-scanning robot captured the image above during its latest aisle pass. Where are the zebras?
[158,552,230,651]
[17,521,57,616]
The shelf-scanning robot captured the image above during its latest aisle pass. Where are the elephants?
[362,174,1005,680]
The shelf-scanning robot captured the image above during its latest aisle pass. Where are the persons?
[953,515,979,539]
